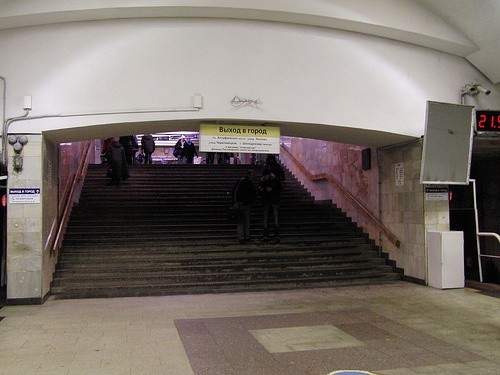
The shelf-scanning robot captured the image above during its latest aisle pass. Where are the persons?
[258,168,281,242]
[233,169,257,244]
[262,154,285,182]
[205,152,234,165]
[173,139,198,164]
[101,132,155,188]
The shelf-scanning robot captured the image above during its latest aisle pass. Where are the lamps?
[12,153,23,173]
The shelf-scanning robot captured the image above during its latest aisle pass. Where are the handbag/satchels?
[122,168,129,180]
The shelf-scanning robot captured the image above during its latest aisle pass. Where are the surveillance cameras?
[476,85,492,95]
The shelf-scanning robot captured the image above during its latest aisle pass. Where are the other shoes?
[239,238,251,245]
[262,234,271,241]
[275,235,281,243]
